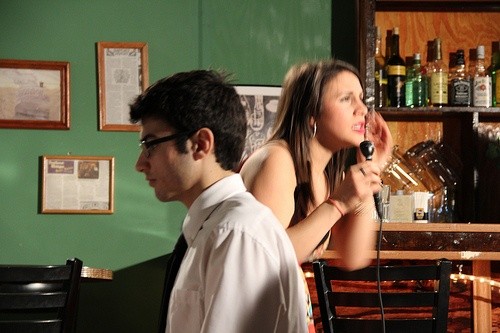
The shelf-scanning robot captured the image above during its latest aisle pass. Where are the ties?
[158,232,188,333]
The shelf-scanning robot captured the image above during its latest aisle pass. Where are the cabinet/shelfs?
[330,0,500,238]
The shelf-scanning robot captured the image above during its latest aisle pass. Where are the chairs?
[311,258,451,333]
[1,257,83,333]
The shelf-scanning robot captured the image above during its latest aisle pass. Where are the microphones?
[359,141,384,218]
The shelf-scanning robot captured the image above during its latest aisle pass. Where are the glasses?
[139,132,180,158]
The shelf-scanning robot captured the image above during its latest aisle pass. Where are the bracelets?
[327,198,345,216]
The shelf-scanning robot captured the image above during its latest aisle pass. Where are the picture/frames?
[95,40,148,133]
[0,59,71,129]
[233,85,287,168]
[41,153,113,216]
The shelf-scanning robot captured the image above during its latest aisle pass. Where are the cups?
[374,184,392,223]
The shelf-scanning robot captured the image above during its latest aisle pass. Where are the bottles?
[449,48,471,107]
[374,26,388,108]
[425,37,450,108]
[405,51,429,108]
[486,41,500,108]
[471,44,492,108]
[385,26,408,107]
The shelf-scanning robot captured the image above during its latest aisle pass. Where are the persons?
[129,68,310,333]
[157,58,394,333]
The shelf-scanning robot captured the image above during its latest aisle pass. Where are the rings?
[359,167,367,176]
